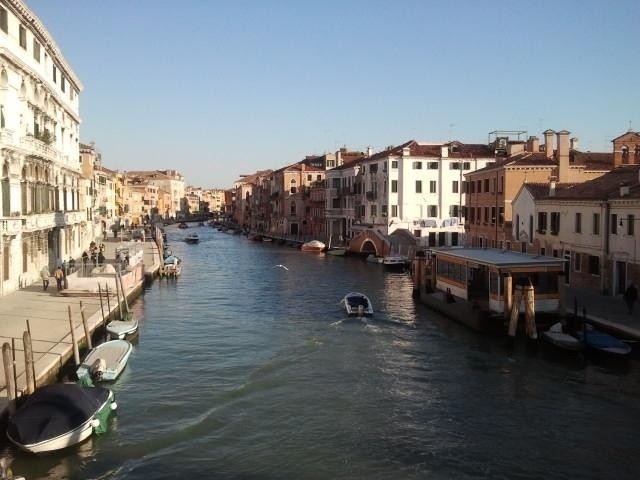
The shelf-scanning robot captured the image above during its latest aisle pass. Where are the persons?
[53,266,63,291]
[63,259,69,276]
[68,256,76,275]
[81,240,107,268]
[40,265,51,291]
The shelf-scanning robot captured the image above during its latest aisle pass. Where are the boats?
[411,243,634,364]
[344,291,375,316]
[382,256,405,272]
[5,382,118,455]
[184,231,199,243]
[75,339,133,382]
[179,221,189,228]
[105,319,139,342]
[327,249,347,257]
[162,255,182,276]
